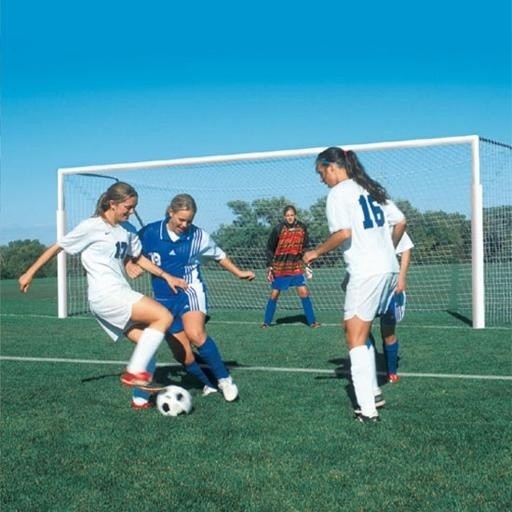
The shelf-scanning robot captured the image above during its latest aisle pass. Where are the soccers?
[156,385,193,417]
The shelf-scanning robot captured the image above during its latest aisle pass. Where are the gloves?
[305,263,314,280]
[266,267,273,283]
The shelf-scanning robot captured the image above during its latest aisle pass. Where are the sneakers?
[130,399,155,409]
[201,385,218,396]
[216,372,239,402]
[386,373,400,383]
[353,394,386,422]
[119,371,166,393]
[311,321,320,329]
[261,323,269,329]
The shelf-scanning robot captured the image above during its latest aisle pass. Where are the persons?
[19,183,187,410]
[125,194,256,402]
[301,147,415,423]
[259,205,320,329]
